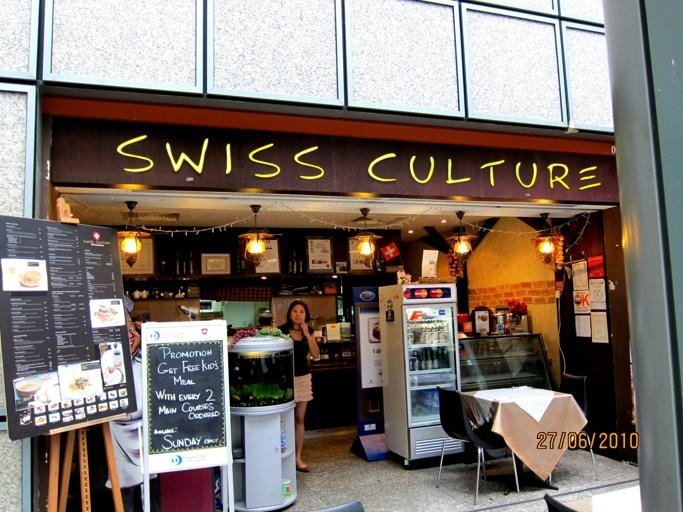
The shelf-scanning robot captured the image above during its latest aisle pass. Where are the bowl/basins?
[15,377,42,400]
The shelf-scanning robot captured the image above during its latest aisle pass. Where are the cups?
[111,351,122,364]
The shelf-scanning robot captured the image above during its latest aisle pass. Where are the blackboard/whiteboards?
[0,214,138,444]
[141,319,233,474]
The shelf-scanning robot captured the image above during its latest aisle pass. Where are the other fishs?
[232,394,241,401]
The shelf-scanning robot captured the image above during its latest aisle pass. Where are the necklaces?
[291,328,301,331]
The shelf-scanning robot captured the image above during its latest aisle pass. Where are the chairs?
[435,373,601,506]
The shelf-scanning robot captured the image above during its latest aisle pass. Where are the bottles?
[311,259,328,265]
[408,322,452,344]
[419,390,439,417]
[410,347,447,370]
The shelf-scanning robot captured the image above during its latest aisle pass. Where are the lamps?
[118,200,149,270]
[349,207,383,258]
[445,210,478,257]
[237,205,279,268]
[531,212,563,263]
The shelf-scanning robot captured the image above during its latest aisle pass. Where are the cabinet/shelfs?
[114,225,401,302]
[458,331,557,465]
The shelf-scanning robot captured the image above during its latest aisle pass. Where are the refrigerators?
[377,281,467,462]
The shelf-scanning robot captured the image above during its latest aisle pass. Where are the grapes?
[230,326,289,348]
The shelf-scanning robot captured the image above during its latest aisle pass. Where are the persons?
[104,296,157,489]
[276,300,320,470]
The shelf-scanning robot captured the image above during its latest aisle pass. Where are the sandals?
[298,466,312,472]
